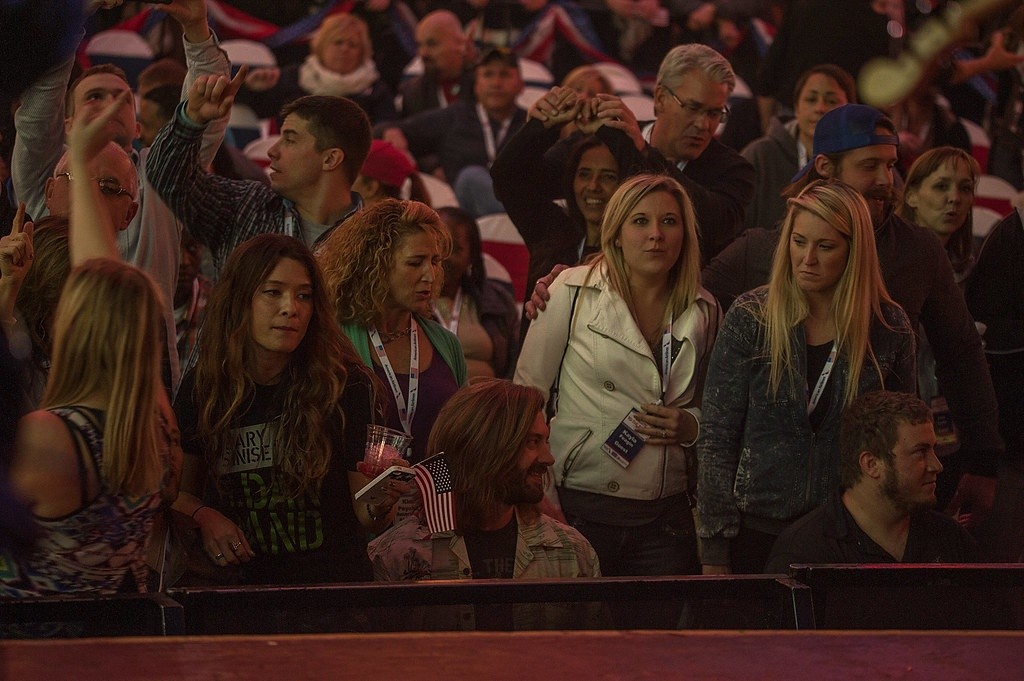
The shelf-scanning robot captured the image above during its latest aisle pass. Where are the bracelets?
[366,504,394,520]
[193,504,208,517]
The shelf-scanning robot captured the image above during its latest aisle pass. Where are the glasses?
[54,172,134,200]
[659,83,732,125]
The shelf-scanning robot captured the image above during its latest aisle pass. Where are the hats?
[358,139,415,189]
[139,59,186,97]
[789,103,899,180]
[474,46,518,68]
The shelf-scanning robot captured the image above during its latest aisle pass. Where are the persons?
[489,1,1024,630]
[0,0,619,639]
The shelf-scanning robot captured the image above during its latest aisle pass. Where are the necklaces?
[376,326,412,343]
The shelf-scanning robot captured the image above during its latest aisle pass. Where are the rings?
[661,428,667,437]
[230,540,241,551]
[216,553,224,559]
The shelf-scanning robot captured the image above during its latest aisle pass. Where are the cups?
[362,423,414,479]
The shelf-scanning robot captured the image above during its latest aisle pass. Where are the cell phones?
[354,466,416,504]
